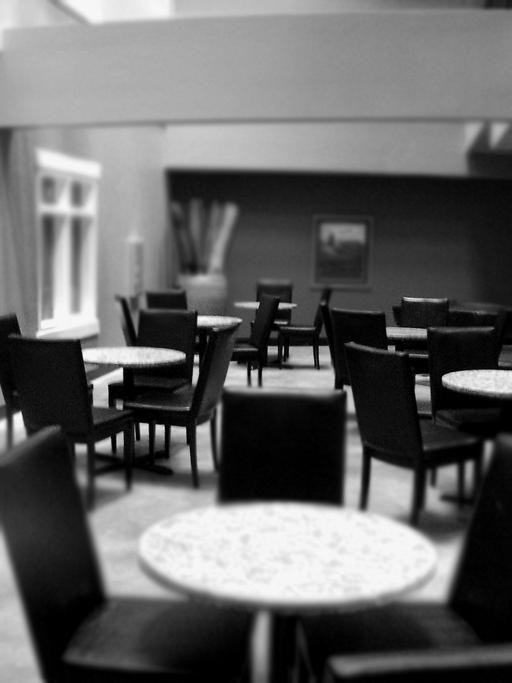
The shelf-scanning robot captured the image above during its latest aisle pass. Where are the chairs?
[2,426,250,680]
[0,277,512,495]
[345,342,485,526]
[8,334,136,508]
[326,434,512,681]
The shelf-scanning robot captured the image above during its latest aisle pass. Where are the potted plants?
[309,211,376,291]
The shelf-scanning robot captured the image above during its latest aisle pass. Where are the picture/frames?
[309,211,376,291]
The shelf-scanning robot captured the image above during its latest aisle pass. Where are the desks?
[137,500,440,682]
